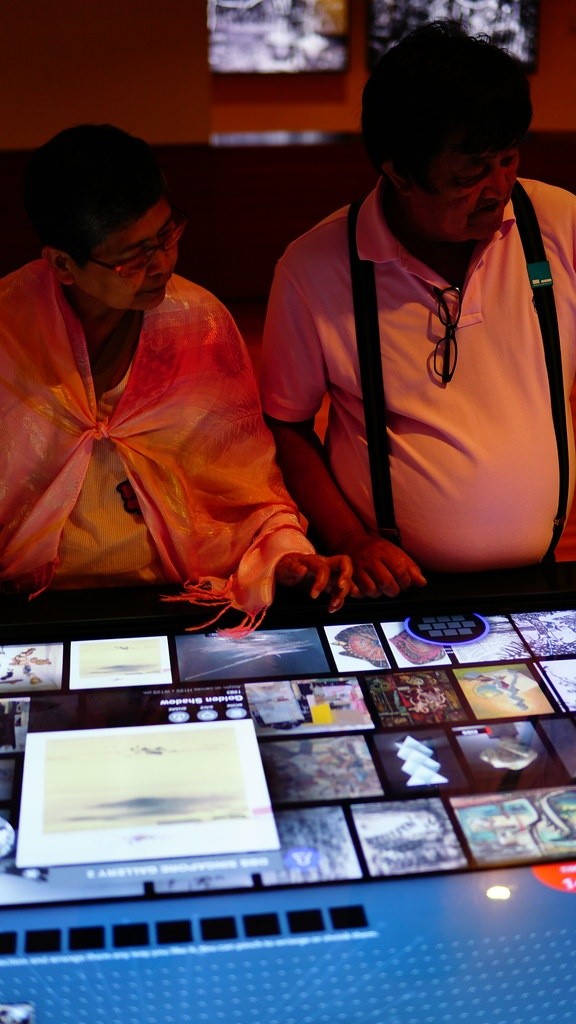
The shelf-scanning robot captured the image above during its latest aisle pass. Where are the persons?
[0,123,359,640]
[257,17,576,600]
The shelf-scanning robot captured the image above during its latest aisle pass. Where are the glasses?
[65,206,190,277]
[434,287,461,383]
